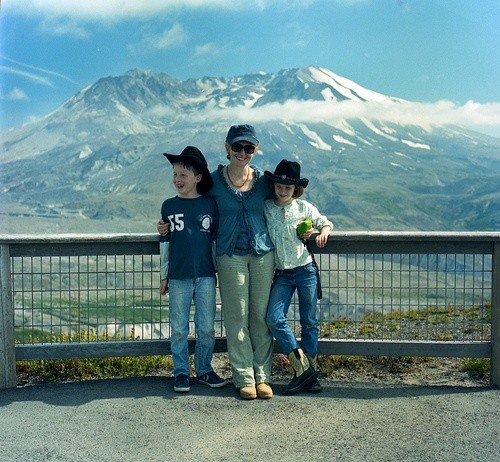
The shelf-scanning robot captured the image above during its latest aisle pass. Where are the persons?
[264,157,334,396]
[157,123,314,400]
[159,145,227,391]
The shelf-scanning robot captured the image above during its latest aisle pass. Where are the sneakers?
[173,374,191,393]
[196,370,228,387]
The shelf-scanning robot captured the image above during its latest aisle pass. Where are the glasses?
[230,143,256,154]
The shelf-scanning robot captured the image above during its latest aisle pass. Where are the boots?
[280,348,318,394]
[303,352,323,392]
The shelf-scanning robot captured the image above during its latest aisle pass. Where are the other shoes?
[239,385,258,399]
[257,383,274,399]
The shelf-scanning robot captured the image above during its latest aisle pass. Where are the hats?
[163,146,214,195]
[263,159,309,189]
[225,124,259,146]
